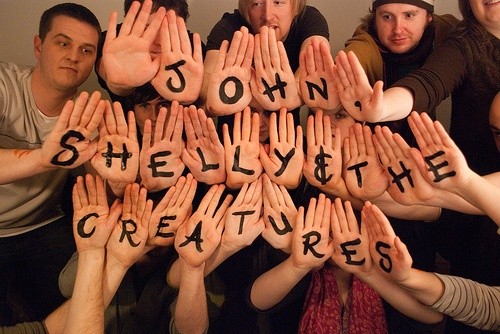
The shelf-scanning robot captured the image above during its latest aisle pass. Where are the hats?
[372,0,434,13]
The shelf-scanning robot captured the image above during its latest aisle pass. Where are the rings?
[344,85,351,87]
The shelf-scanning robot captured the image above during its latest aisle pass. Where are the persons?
[0,0,500,334]
